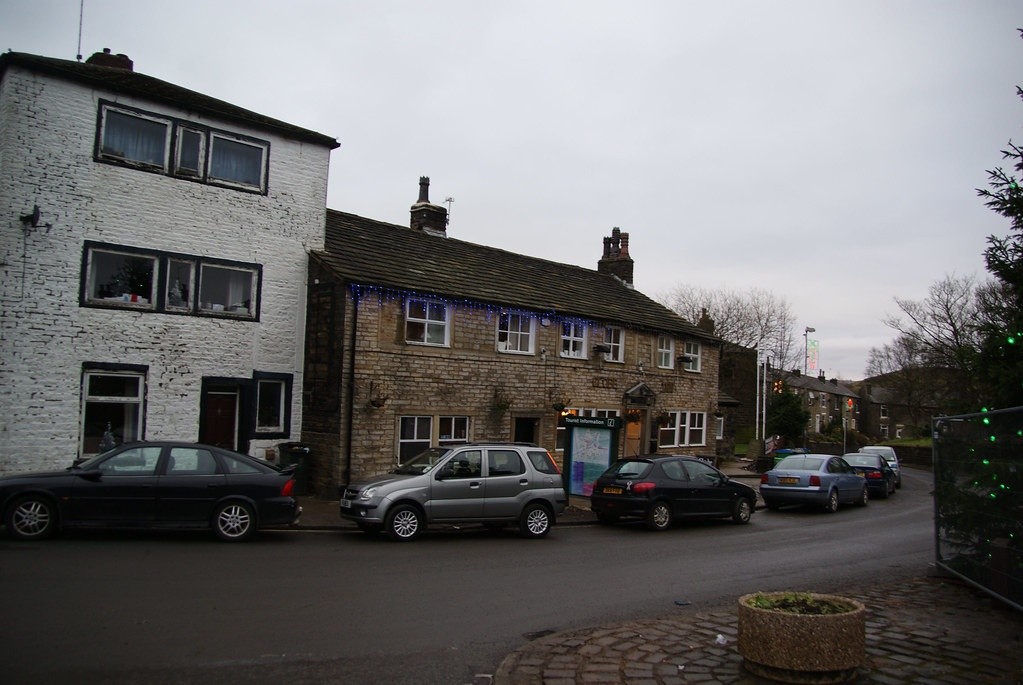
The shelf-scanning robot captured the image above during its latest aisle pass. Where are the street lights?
[804,326,816,448]
[754,337,776,441]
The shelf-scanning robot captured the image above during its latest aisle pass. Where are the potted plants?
[738,591,866,670]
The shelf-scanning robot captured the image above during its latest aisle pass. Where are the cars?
[759,453,870,513]
[0,439,305,542]
[842,445,902,500]
[590,453,757,531]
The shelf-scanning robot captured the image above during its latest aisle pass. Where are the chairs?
[453,456,515,478]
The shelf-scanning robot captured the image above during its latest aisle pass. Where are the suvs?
[341,440,570,543]
